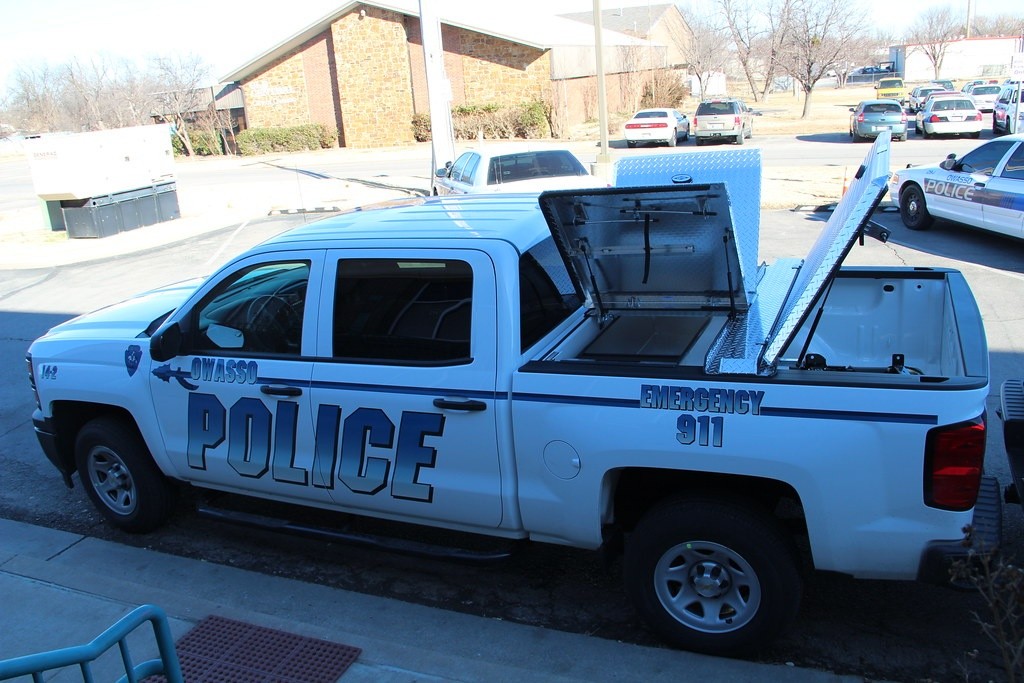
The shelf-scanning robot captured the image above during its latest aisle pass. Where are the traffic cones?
[838,164,851,198]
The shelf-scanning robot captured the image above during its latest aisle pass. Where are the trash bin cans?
[61,179,181,239]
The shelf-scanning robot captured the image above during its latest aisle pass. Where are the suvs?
[992,84,1024,134]
[874,75,910,105]
[692,98,758,145]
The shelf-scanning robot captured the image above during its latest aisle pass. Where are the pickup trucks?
[25,133,1024,657]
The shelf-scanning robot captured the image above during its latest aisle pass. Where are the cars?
[913,94,984,139]
[433,142,602,204]
[622,108,691,147]
[907,77,1024,112]
[850,101,911,143]
[890,134,1024,239]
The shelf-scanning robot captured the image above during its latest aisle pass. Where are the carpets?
[139,615,362,683]
